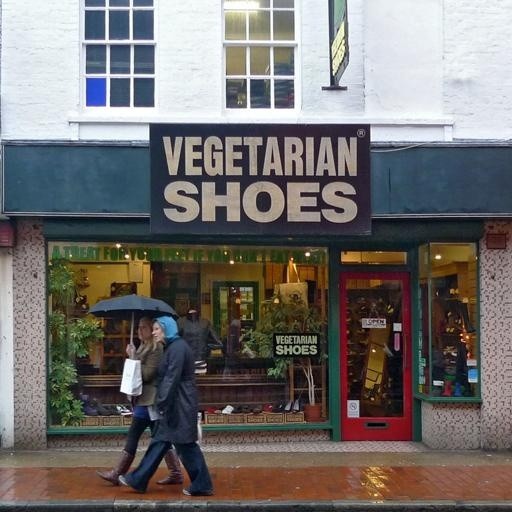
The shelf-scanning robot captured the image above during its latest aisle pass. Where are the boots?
[447,279,453,297]
[156,449,184,484]
[94,449,135,485]
[463,312,474,332]
[456,342,467,379]
[433,380,472,396]
[79,393,112,416]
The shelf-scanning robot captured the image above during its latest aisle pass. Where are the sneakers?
[105,404,134,416]
[119,474,146,492]
[183,487,213,495]
[207,402,273,414]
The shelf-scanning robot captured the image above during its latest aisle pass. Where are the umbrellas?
[87,293,180,345]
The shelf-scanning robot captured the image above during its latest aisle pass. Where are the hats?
[153,316,178,338]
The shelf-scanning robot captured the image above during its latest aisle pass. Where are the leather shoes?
[273,399,301,413]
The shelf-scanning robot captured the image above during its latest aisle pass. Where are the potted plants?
[239,289,332,428]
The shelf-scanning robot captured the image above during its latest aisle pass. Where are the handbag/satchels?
[119,359,143,397]
[279,283,309,308]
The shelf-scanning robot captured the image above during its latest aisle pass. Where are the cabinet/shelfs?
[98,296,140,375]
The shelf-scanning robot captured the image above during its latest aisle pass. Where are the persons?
[177,309,222,374]
[117,315,216,496]
[94,315,185,486]
